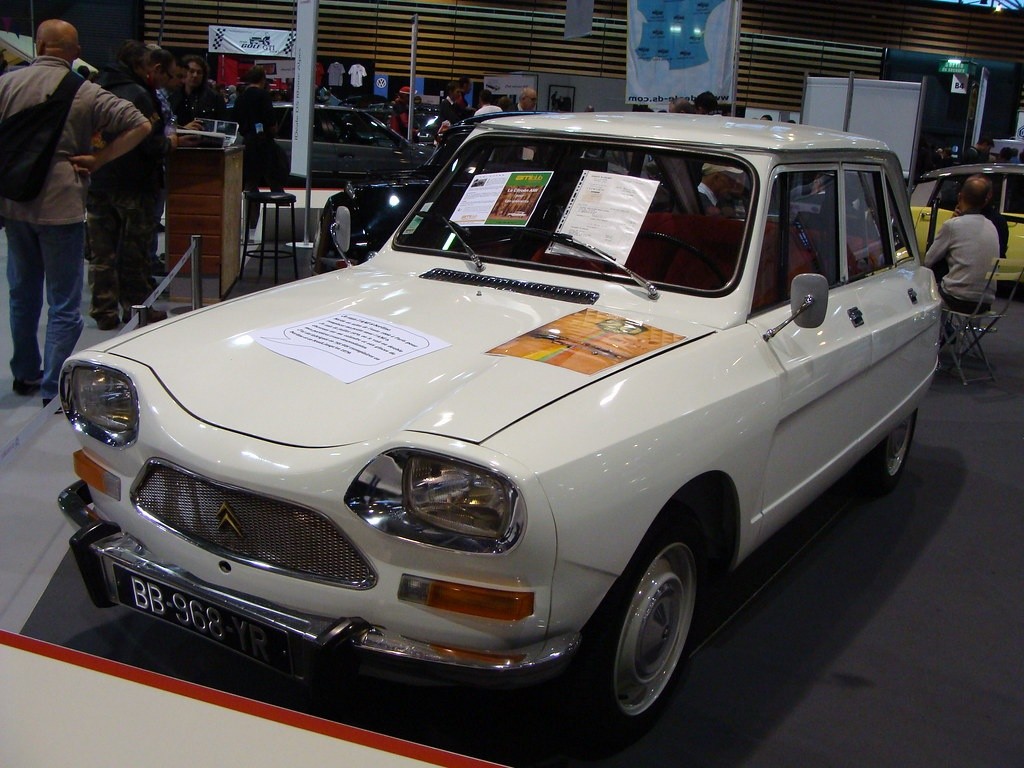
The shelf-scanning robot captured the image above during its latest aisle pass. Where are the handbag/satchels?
[0,69,86,202]
[395,114,408,138]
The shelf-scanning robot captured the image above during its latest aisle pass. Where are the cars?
[56,113,944,731]
[905,161,1024,282]
[320,111,551,260]
[225,104,431,177]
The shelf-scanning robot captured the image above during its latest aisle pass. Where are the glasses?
[166,70,175,81]
[456,90,463,93]
[526,96,537,102]
[414,101,421,104]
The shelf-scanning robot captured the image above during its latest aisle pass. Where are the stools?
[238,191,299,287]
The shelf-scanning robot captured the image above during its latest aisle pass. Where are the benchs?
[532,212,812,312]
[804,227,885,276]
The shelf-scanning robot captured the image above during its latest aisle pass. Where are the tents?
[0,30,99,74]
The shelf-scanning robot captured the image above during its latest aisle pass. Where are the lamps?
[995,2,1001,12]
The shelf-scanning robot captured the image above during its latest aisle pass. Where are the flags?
[564,0,594,38]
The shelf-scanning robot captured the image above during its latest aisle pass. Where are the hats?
[400,86,410,95]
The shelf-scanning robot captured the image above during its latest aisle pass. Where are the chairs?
[938,257,1024,389]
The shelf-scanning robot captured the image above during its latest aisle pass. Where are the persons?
[0,19,152,408]
[905,133,1024,211]
[0,38,178,331]
[226,68,291,243]
[924,175,1009,355]
[644,90,835,232]
[387,78,537,147]
[166,57,236,129]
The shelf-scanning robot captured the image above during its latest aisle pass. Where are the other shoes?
[14,369,45,396]
[122,305,168,324]
[146,261,166,277]
[143,275,169,300]
[157,223,165,232]
[938,322,957,344]
[98,316,120,330]
[42,399,63,413]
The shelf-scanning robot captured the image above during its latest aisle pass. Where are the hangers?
[334,60,339,65]
